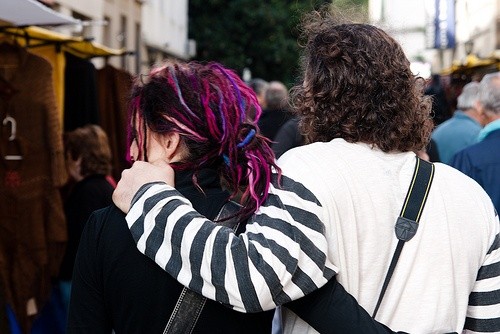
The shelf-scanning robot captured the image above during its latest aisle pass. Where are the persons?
[0,125,128,334]
[111,22,500,334]
[66,61,405,334]
[408,45,499,215]
[240,76,310,161]
